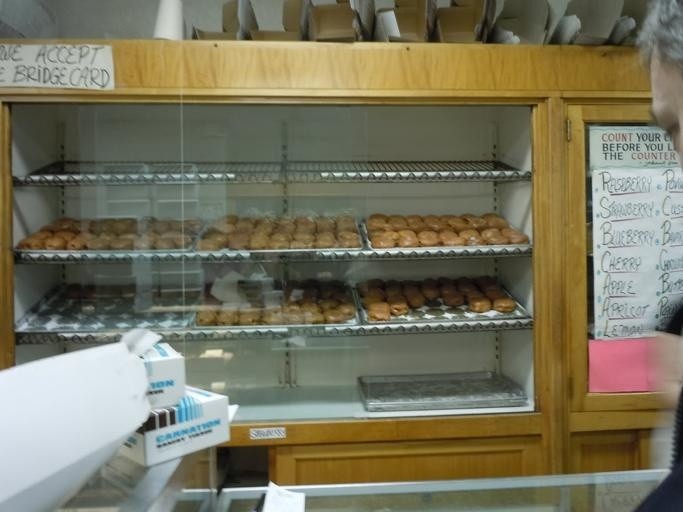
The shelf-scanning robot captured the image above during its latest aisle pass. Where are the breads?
[196,276,517,326]
[17,212,529,251]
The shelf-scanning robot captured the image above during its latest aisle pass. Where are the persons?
[628,1,682,511]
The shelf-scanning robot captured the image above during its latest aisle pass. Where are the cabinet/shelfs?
[0,35,682,511]
